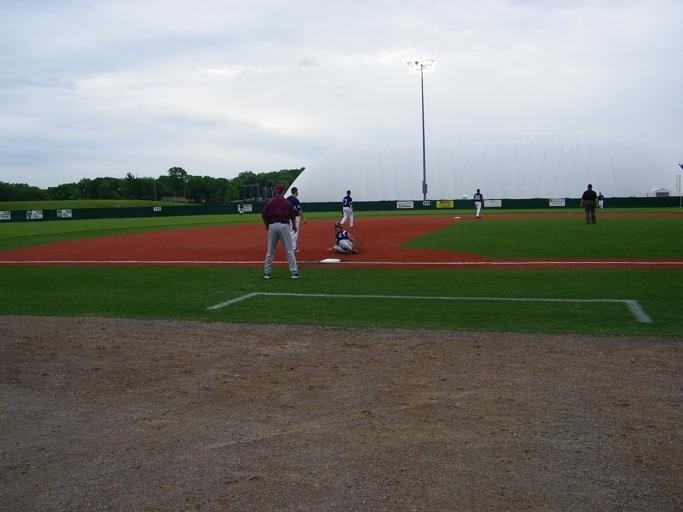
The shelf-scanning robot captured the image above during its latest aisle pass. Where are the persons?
[473,188,485,217]
[579,183,598,225]
[598,192,605,209]
[325,222,359,254]
[238,200,245,216]
[340,189,355,229]
[261,183,303,279]
[287,187,308,252]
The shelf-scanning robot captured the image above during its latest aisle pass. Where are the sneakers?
[264,273,272,279]
[291,274,302,279]
